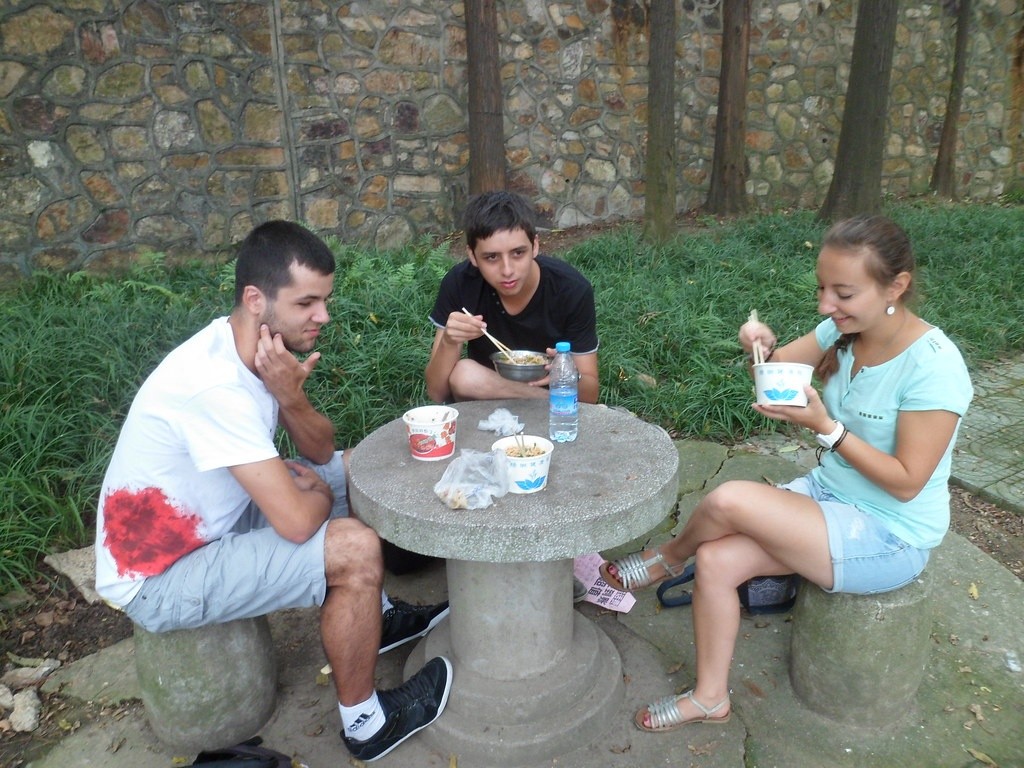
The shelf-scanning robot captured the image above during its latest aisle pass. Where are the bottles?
[548,342,578,443]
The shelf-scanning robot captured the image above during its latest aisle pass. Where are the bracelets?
[578,371,581,380]
[751,350,774,365]
[815,428,849,467]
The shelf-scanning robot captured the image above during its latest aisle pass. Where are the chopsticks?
[462,307,519,366]
[510,428,526,457]
[747,308,764,363]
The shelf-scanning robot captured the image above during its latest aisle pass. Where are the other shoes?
[572,573,588,602]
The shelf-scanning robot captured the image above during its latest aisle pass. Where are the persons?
[95,218,454,761]
[423,192,599,402]
[597,217,975,733]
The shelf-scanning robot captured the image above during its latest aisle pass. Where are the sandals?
[600,547,688,590]
[635,689,731,732]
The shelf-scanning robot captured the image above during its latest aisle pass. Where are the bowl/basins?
[752,362,814,408]
[489,350,554,383]
[402,405,459,462]
[491,434,555,495]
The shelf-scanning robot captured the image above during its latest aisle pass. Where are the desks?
[348,399,680,762]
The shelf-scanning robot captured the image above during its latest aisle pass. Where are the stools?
[789,579,935,729]
[133,613,279,754]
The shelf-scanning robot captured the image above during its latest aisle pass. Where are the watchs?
[816,420,845,450]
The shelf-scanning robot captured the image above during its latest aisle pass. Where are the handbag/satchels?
[657,562,800,615]
[180,736,291,768]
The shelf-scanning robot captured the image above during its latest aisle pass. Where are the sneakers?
[339,654,453,763]
[377,594,451,653]
